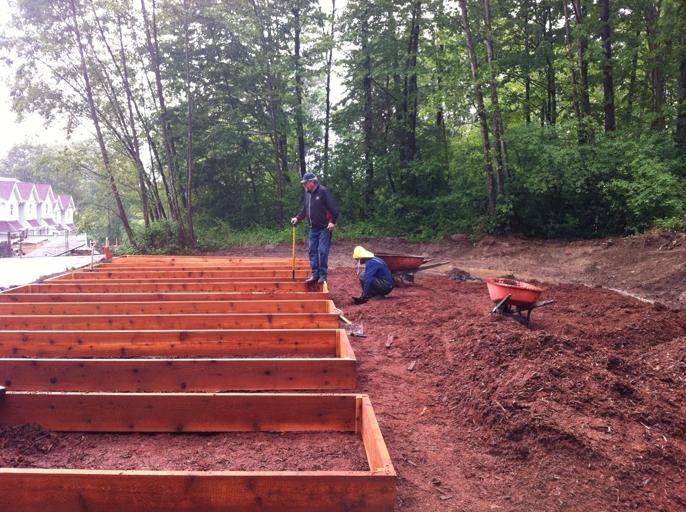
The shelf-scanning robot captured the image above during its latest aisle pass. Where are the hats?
[300,173,317,183]
[353,246,374,258]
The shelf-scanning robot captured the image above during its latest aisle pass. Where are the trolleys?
[369,249,452,284]
[484,274,559,329]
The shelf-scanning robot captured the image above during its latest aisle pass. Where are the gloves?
[290,218,298,224]
[326,222,334,232]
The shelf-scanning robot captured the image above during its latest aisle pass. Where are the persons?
[353,246,394,303]
[291,172,338,284]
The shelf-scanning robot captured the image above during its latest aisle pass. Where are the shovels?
[339,314,363,337]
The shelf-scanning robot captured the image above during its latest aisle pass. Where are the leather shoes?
[306,276,326,283]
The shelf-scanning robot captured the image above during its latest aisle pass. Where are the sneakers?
[372,294,384,300]
